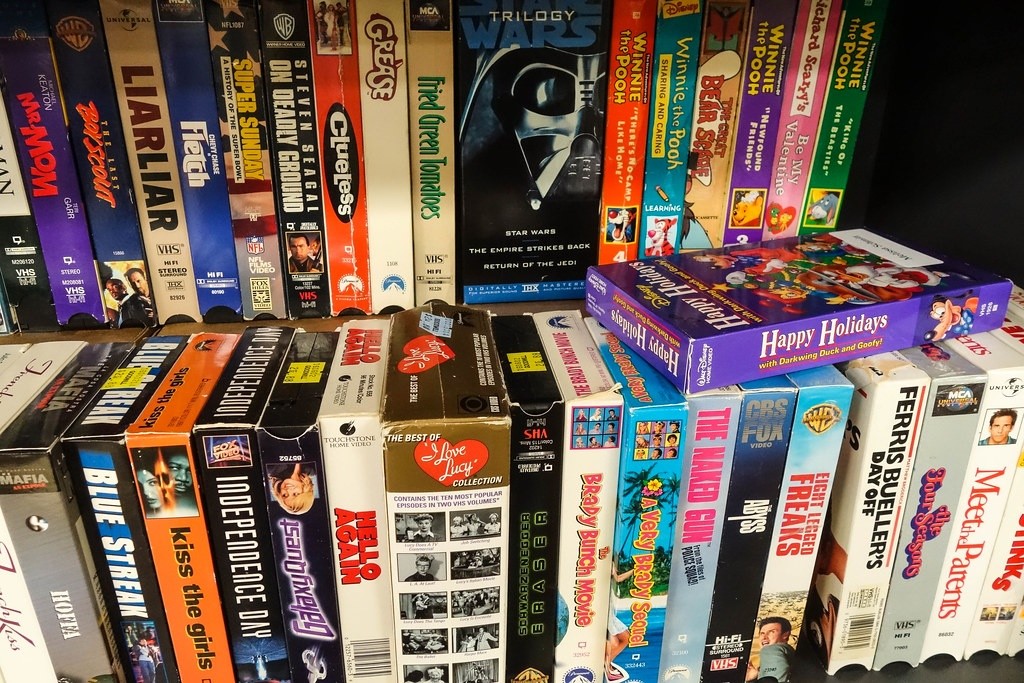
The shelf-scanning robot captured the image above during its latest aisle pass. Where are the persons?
[404,554,435,581]
[635,422,680,460]
[128,625,156,683]
[404,513,445,542]
[105,268,154,326]
[605,563,633,680]
[450,512,501,538]
[266,462,318,515]
[458,627,498,652]
[411,593,434,619]
[136,452,193,511]
[744,617,796,682]
[575,408,619,448]
[977,408,1018,446]
[288,233,322,272]
